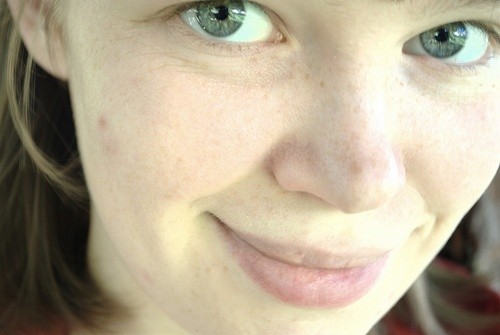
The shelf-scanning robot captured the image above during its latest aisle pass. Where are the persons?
[1,0,500,335]
